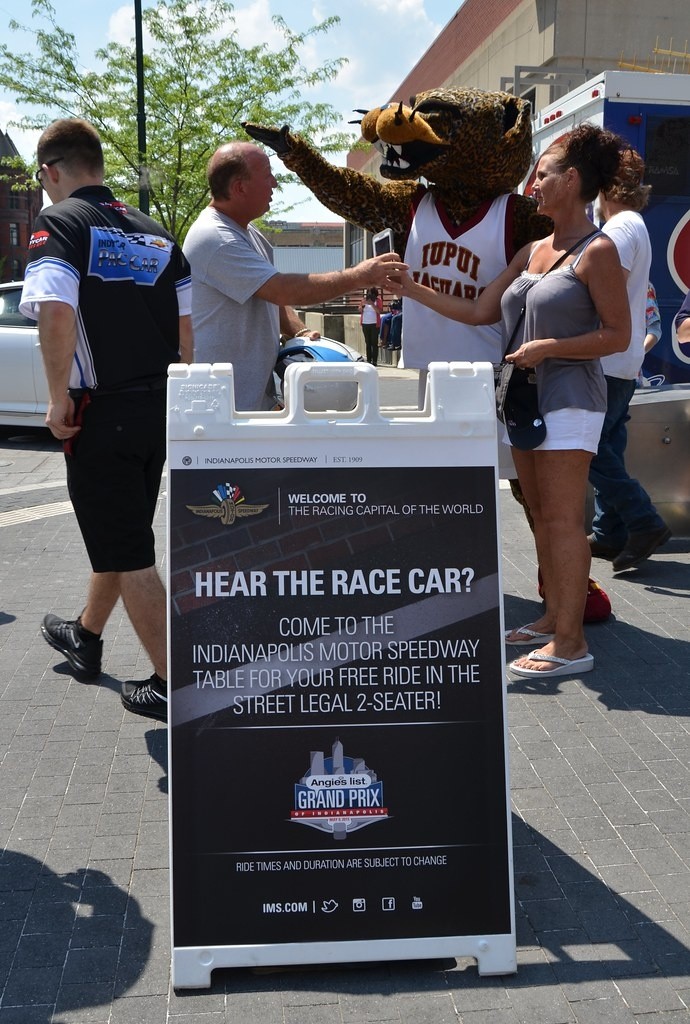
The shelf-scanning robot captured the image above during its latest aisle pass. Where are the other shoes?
[587,533,624,560]
[613,524,672,571]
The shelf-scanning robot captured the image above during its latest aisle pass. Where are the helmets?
[265,336,364,412]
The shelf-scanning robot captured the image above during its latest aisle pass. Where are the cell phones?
[372,226,396,277]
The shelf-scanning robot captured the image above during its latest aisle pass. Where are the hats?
[496,360,546,451]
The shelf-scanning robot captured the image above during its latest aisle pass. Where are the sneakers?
[41,613,103,678]
[121,673,167,724]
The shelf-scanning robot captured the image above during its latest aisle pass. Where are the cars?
[1,280,74,435]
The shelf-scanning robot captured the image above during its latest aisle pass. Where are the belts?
[68,379,165,398]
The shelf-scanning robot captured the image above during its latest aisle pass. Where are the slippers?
[509,649,594,678]
[504,623,556,645]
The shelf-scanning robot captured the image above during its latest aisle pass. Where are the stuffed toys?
[241,87,611,624]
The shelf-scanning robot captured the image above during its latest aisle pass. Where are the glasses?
[36,157,63,190]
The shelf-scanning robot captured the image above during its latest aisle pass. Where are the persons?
[675,291,690,343]
[636,281,662,387]
[181,142,410,411]
[587,150,672,578]
[19,119,194,717]
[383,124,633,678]
[359,288,402,366]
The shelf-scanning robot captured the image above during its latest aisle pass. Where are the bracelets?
[295,328,311,337]
